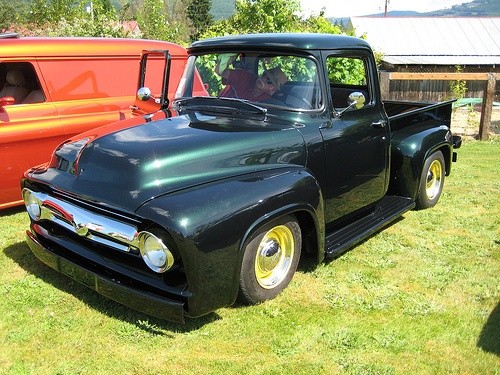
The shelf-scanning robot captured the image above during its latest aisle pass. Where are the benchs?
[273,81,369,109]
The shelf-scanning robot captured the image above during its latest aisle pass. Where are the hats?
[265,66,288,91]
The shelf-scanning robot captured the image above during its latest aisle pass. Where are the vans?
[0,31,211,210]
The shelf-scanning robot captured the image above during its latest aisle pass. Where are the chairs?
[0,69,28,105]
[21,90,44,104]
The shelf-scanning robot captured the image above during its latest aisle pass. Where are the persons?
[215,53,287,102]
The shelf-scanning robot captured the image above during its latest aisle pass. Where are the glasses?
[262,73,272,84]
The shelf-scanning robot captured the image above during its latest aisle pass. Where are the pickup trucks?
[20,33,462,325]
